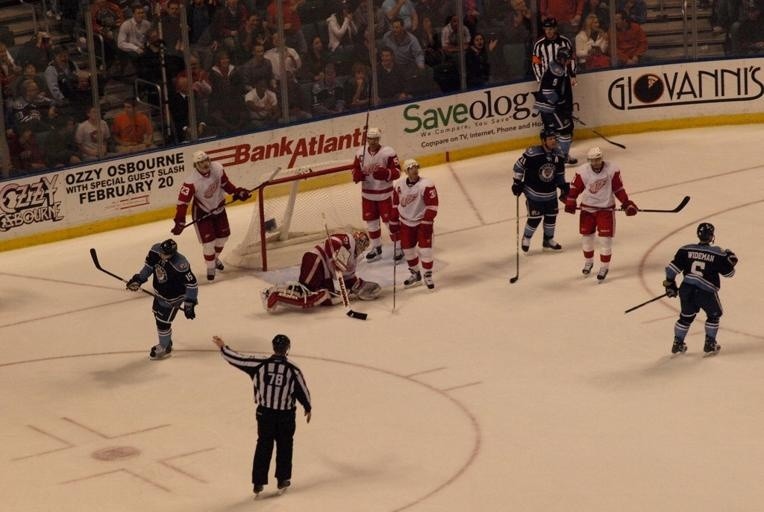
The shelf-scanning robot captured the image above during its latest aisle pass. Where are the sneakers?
[543,239,562,249]
[522,235,530,252]
[149,343,172,357]
[254,484,264,493]
[215,257,224,270]
[278,480,290,489]
[564,154,578,164]
[704,339,721,353]
[597,267,608,279]
[267,286,278,311]
[367,247,434,289]
[582,263,593,273]
[672,336,687,353]
[207,267,216,279]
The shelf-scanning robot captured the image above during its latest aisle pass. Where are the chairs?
[30,0,610,177]
[729,21,764,57]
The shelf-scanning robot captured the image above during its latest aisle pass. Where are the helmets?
[403,159,419,175]
[367,128,382,139]
[557,47,571,65]
[158,239,177,261]
[587,146,604,166]
[542,17,560,40]
[273,335,291,356]
[698,223,715,243]
[192,150,211,175]
[351,230,369,256]
[540,129,556,139]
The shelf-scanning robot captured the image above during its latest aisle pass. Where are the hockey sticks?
[576,197,690,212]
[185,166,281,228]
[90,249,185,311]
[361,64,372,168]
[324,223,367,320]
[572,115,627,150]
[390,240,396,313]
[511,194,519,283]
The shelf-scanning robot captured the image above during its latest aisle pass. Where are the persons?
[258,230,381,312]
[125,239,199,361]
[564,146,638,282]
[386,158,440,291]
[0,1,763,172]
[169,151,250,283]
[511,126,571,252]
[351,127,403,264]
[663,221,738,354]
[210,333,313,498]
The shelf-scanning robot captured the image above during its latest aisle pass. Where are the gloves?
[171,217,185,235]
[352,278,381,300]
[664,280,677,297]
[512,178,524,196]
[183,299,198,320]
[621,200,638,215]
[353,171,365,183]
[126,274,147,291]
[419,222,433,237]
[373,167,390,179]
[389,220,400,233]
[233,187,252,200]
[726,248,739,264]
[560,183,576,214]
